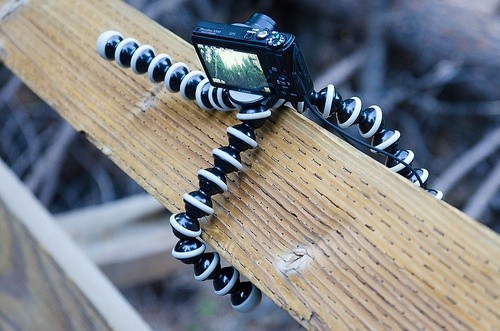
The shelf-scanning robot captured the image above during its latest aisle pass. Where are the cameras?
[192,13,313,103]
